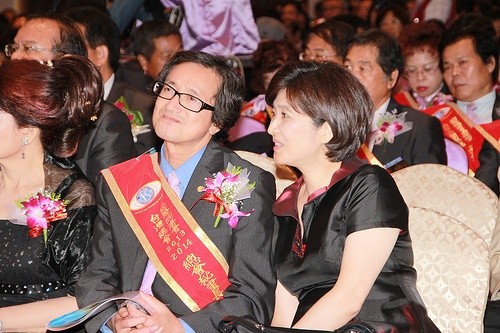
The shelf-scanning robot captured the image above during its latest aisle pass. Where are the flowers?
[15,189,72,250]
[432,92,455,106]
[196,162,256,228]
[372,109,405,145]
[114,95,144,125]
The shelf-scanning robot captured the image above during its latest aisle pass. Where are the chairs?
[388,163,500,333]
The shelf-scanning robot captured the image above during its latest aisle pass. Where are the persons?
[0,51,95,333]
[75,49,277,333]
[1,0,500,197]
[265,60,443,333]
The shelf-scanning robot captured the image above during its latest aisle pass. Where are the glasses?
[5,44,67,57]
[404,63,439,79]
[153,81,214,112]
[300,52,338,61]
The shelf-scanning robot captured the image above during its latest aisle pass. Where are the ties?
[140,171,181,297]
[467,103,482,126]
